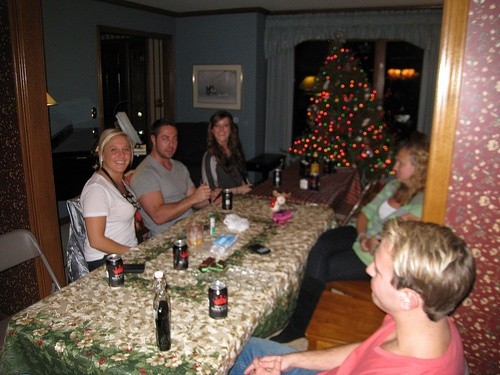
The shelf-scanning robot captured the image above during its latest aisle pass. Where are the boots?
[269,274,323,343]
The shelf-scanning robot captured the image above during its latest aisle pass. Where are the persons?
[79,129,141,273]
[201,111,253,196]
[129,122,222,236]
[226,214,476,375]
[269,138,430,342]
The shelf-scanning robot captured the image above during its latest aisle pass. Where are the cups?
[299,178,308,190]
[187,224,203,245]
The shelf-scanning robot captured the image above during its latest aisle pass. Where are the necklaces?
[101,167,141,209]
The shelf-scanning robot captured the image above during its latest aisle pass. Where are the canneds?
[106,254,125,287]
[208,281,229,320]
[221,189,233,210]
[308,173,321,192]
[172,239,189,271]
[272,169,282,188]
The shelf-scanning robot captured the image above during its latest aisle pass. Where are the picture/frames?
[192,64,242,111]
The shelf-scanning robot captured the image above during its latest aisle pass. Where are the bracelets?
[356,232,365,240]
[236,185,238,195]
[376,232,382,243]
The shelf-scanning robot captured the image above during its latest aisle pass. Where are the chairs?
[305,172,388,352]
[0,197,89,349]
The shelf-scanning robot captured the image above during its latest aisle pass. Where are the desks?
[246,161,362,224]
[0,198,338,375]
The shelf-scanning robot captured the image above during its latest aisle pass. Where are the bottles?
[149,271,172,351]
[309,151,321,192]
